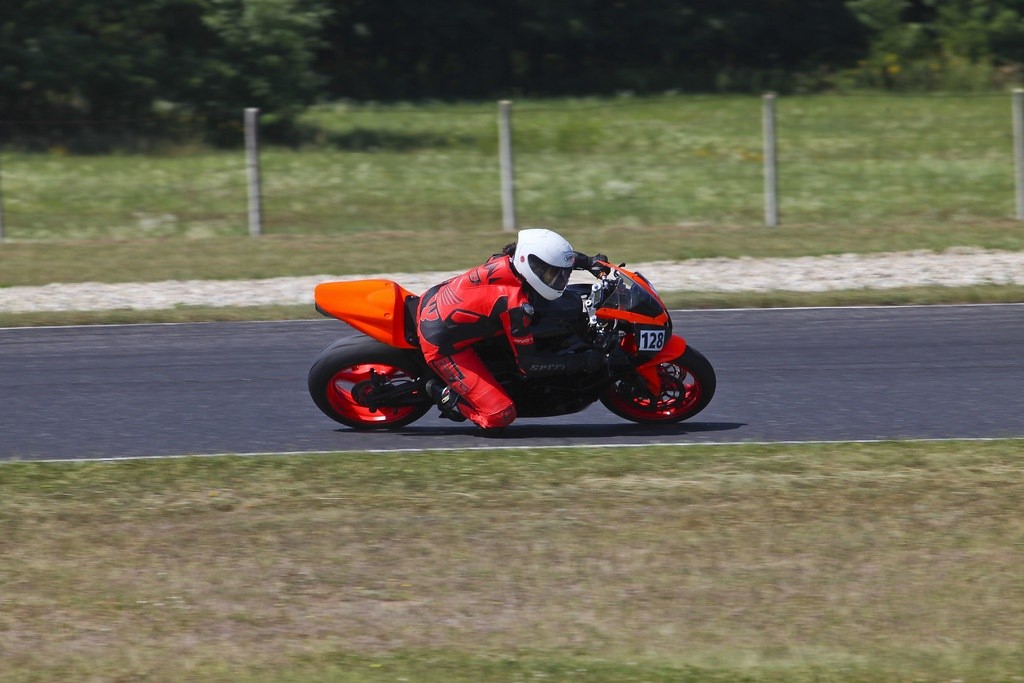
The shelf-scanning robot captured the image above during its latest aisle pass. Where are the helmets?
[512,229,574,301]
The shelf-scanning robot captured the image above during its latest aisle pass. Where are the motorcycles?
[306,262,717,433]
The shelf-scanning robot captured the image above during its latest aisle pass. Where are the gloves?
[575,344,605,374]
[584,254,610,279]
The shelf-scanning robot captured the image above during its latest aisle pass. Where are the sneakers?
[425,379,466,421]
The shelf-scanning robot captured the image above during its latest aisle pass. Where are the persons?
[416,226,611,433]
